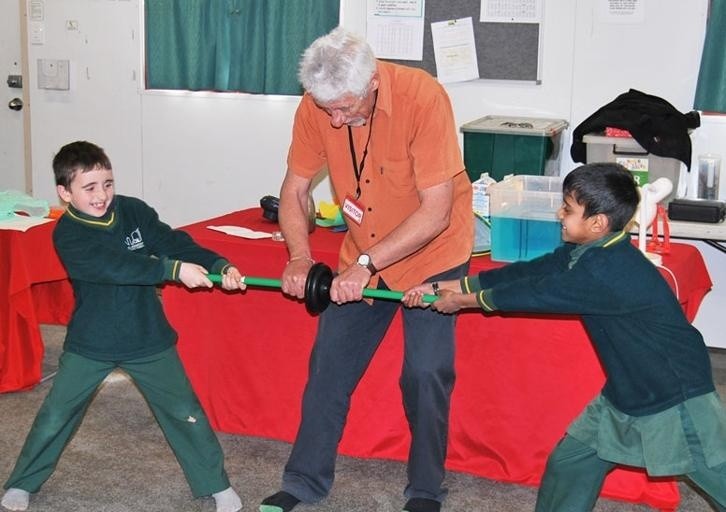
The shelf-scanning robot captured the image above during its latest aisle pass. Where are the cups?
[698,152,721,201]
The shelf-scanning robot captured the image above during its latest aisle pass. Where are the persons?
[254,26,477,512]
[1,136,249,512]
[397,160,726,511]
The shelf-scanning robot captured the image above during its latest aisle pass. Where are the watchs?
[356,253,376,276]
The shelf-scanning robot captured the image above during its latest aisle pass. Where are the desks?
[162,209,713,511]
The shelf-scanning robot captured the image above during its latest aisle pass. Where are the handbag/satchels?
[668,198,726,223]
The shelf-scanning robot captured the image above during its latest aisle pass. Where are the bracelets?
[283,256,317,265]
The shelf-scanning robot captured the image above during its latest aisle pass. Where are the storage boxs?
[458,115,681,262]
